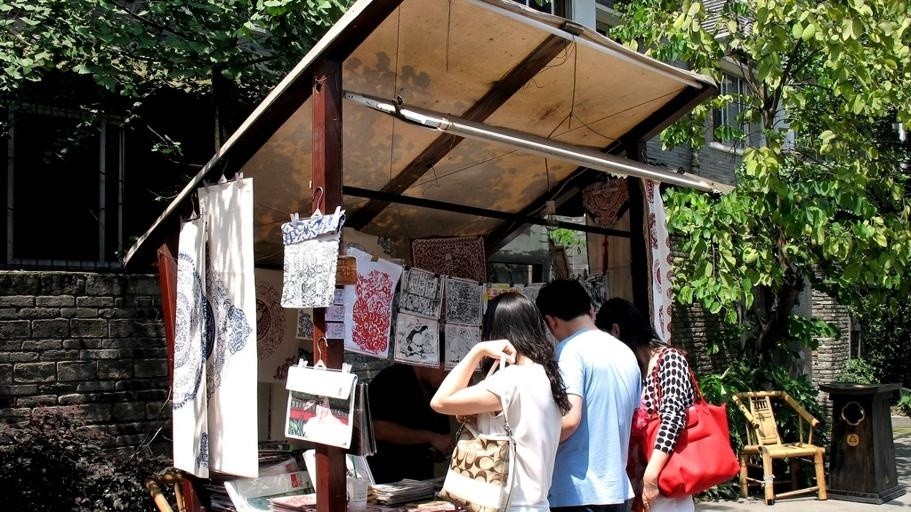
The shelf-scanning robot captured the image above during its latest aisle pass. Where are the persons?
[430,291,576,512]
[592,293,701,512]
[531,276,645,512]
[369,330,471,485]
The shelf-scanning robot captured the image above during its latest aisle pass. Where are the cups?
[345,468,370,511]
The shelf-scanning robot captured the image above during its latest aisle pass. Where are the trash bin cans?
[819,383,907,505]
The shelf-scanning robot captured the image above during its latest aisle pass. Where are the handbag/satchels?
[437,437,516,512]
[641,348,740,497]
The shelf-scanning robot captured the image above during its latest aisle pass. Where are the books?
[286,377,384,459]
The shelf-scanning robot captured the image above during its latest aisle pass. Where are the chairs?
[730,390,828,505]
